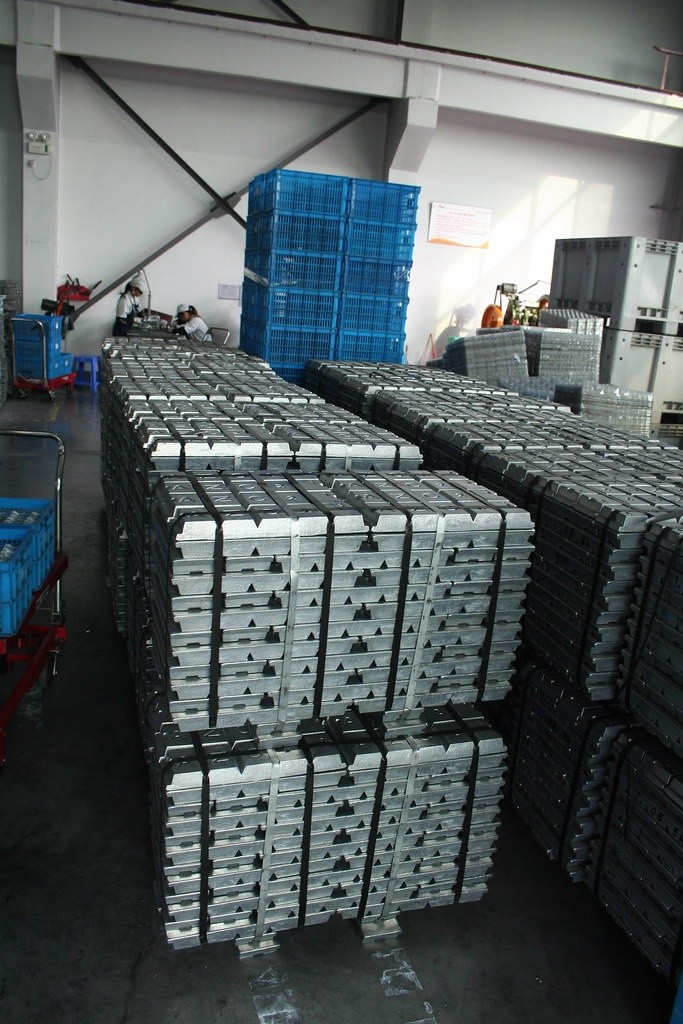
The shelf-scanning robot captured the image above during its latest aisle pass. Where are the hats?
[130,276,146,293]
[175,304,189,318]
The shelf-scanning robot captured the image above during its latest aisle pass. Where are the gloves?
[133,316,142,324]
[166,326,174,332]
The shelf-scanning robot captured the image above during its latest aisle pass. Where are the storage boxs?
[0,168,683,990]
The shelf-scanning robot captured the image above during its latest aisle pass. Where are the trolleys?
[8,317,78,402]
[0,427,70,772]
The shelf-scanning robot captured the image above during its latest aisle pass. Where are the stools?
[75,354,100,393]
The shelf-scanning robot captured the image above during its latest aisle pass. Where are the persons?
[112,278,144,337]
[176,305,212,342]
